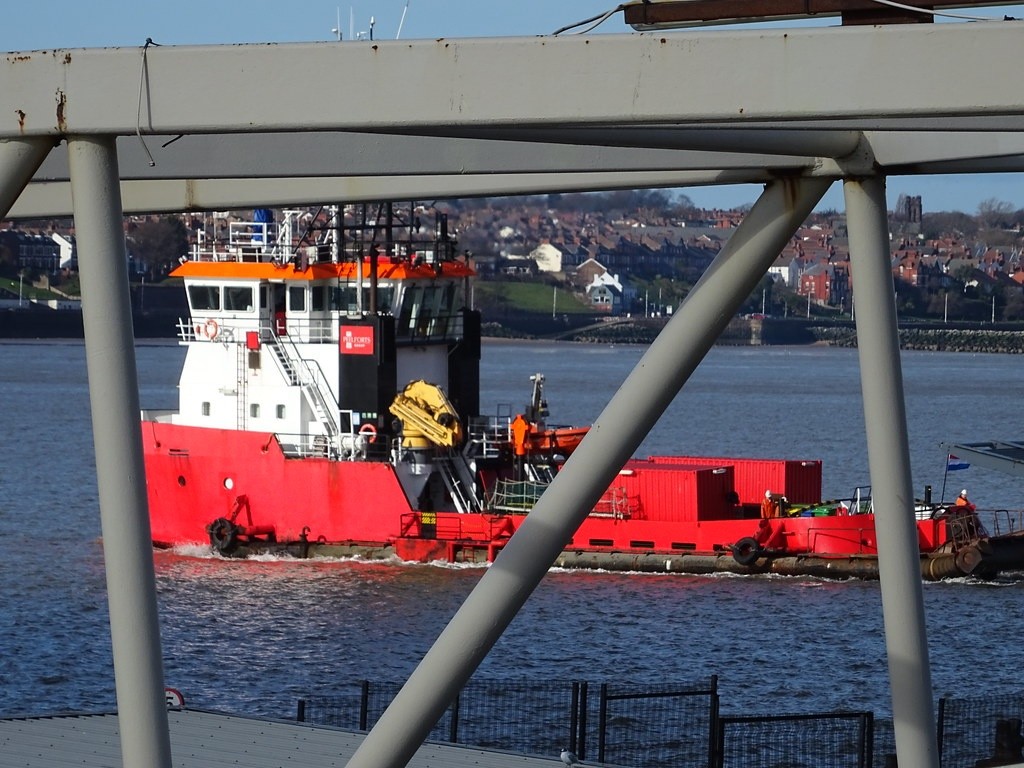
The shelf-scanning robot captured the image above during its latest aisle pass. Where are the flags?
[947,455,971,472]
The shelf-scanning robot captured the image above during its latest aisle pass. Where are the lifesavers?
[731,534,761,566]
[203,320,221,339]
[358,423,377,443]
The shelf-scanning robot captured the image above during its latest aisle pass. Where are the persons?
[955,489,971,507]
[760,489,789,518]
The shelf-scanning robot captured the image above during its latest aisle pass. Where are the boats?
[140,198,995,578]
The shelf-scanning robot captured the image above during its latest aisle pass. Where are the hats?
[765,490,772,498]
[961,489,967,495]
